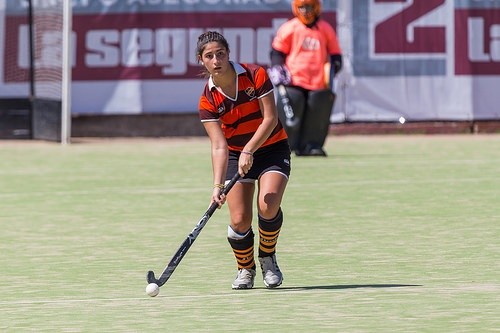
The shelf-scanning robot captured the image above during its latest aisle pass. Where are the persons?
[197,31,291,290]
[268,0,344,157]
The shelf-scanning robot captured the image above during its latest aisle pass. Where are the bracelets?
[241,151,253,155]
[213,183,226,189]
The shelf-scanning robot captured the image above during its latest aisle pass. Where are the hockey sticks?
[147,157,256,287]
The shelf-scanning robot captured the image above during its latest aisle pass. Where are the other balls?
[146,283,159,296]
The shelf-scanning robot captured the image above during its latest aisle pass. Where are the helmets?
[292,0,320,25]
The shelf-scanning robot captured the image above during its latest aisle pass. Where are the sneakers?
[258,251,284,289]
[231,266,256,290]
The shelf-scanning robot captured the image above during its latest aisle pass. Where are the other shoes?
[310,147,326,157]
[295,149,308,156]
